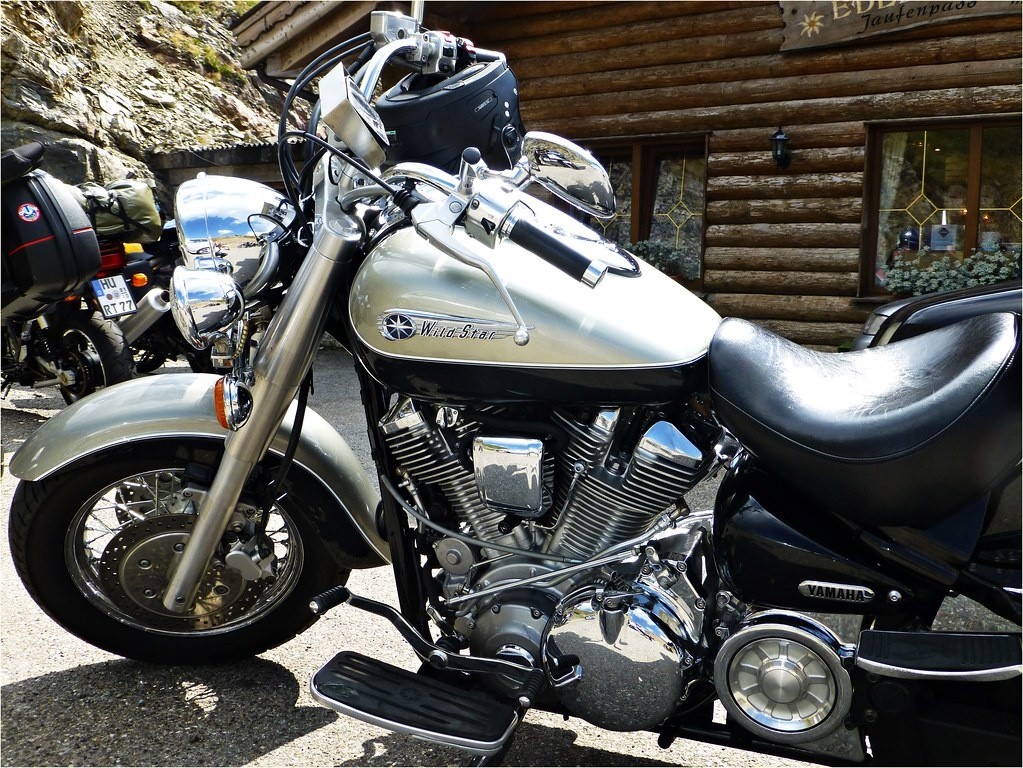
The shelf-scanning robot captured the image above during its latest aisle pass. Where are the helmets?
[378,49,522,172]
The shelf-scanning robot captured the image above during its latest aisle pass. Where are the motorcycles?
[0,139,260,409]
[0,0,1022,767]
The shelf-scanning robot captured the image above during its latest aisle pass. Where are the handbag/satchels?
[70,177,166,245]
